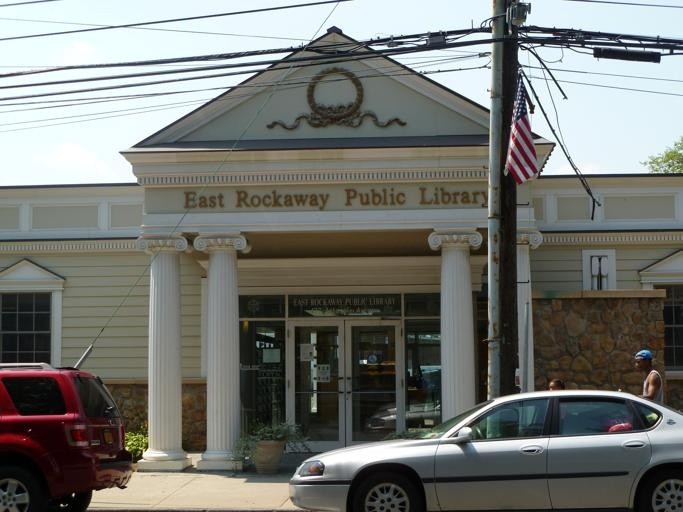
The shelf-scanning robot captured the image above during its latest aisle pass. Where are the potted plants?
[232,422,312,475]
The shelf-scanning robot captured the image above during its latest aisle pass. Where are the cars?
[0,361,137,512]
[364,401,441,431]
[289,390,683,512]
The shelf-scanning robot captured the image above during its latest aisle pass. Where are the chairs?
[496,408,541,435]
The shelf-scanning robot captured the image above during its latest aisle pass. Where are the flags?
[501,74,540,189]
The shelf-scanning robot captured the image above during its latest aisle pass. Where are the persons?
[546,379,567,392]
[631,349,663,417]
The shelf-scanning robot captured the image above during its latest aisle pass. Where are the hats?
[635,349,653,360]
